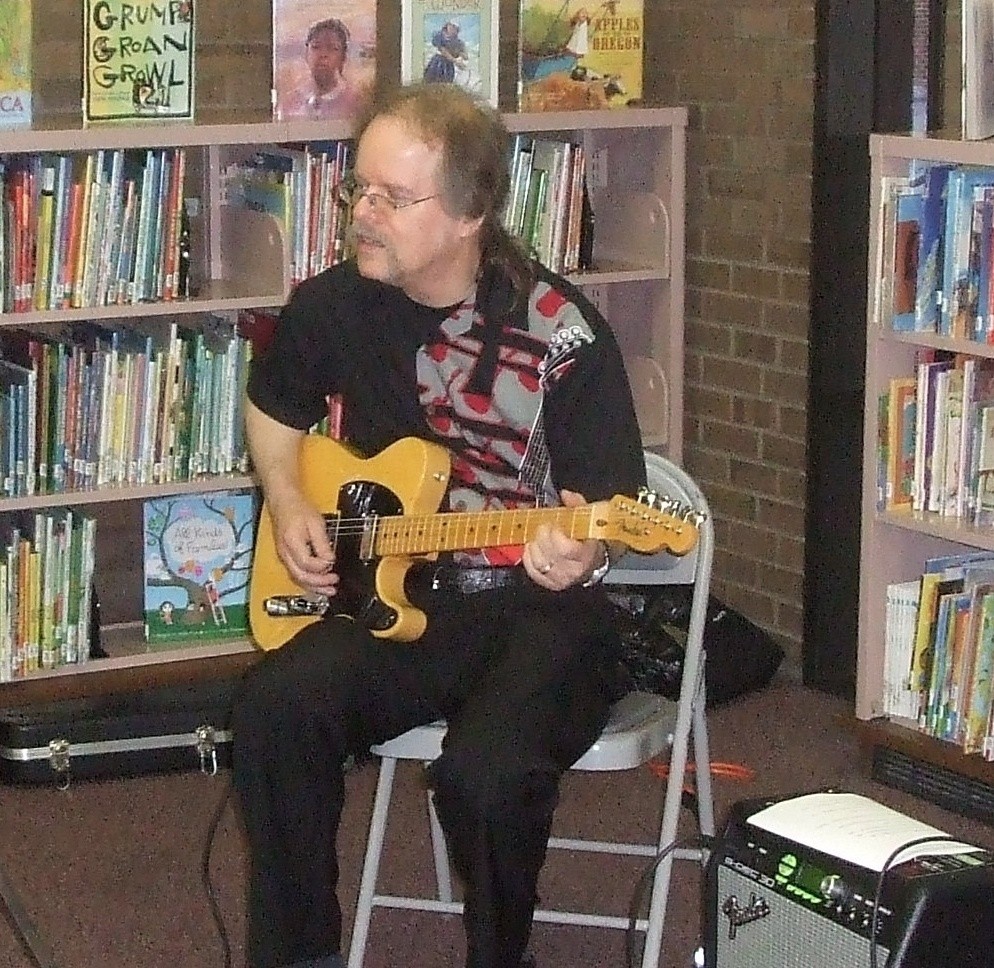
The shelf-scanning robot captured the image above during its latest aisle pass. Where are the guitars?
[244,432,708,654]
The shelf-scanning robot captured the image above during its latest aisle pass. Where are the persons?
[235,90,649,968]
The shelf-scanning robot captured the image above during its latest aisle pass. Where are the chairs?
[346,456,719,968]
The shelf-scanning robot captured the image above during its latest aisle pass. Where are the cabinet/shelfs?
[1,108,690,783]
[858,128,994,839]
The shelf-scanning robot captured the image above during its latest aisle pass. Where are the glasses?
[332,175,444,215]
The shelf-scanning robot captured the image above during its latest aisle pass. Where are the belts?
[412,566,523,593]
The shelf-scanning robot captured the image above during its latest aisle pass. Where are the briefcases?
[3,681,233,790]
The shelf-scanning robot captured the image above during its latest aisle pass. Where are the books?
[878,355,994,528]
[0,507,99,678]
[873,160,994,347]
[499,132,596,274]
[308,392,343,444]
[884,552,994,762]
[961,0,994,142]
[143,489,256,642]
[0,323,252,498]
[0,148,192,315]
[219,141,347,289]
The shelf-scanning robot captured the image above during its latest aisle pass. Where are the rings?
[540,562,554,574]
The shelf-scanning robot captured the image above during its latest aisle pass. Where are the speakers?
[698,785,994,968]
